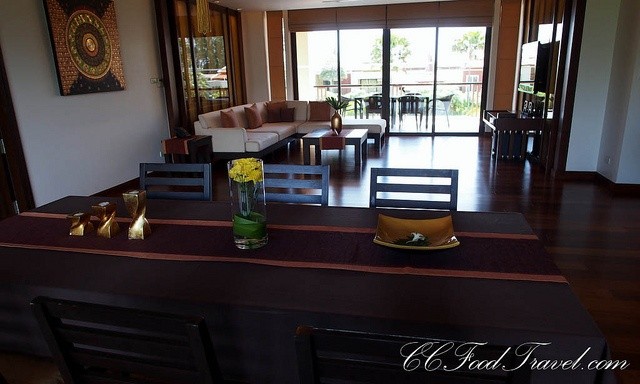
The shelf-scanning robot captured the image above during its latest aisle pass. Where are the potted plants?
[325,96,351,134]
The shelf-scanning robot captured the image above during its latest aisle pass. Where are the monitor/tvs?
[519,40,543,83]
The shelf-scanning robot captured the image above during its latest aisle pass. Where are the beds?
[302,128,368,181]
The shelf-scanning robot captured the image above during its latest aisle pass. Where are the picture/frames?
[44,1,127,97]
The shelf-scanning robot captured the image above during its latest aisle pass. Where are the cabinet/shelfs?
[480,110,551,163]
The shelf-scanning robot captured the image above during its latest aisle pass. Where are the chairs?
[242,164,331,207]
[292,324,532,384]
[370,168,459,213]
[406,92,421,95]
[365,96,383,119]
[398,96,419,132]
[374,93,382,95]
[429,93,455,127]
[29,295,223,382]
[138,163,213,204]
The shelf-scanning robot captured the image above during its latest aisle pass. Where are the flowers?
[228,158,264,217]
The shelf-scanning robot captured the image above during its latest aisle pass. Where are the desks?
[0,196,606,384]
[160,135,213,163]
[354,94,429,129]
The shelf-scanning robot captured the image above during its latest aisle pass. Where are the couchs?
[194,101,388,159]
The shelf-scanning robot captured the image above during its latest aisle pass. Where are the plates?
[373,215,460,250]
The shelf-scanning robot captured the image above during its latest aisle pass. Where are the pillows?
[219,107,240,127]
[266,101,289,122]
[308,100,331,121]
[244,103,263,127]
[280,108,295,122]
